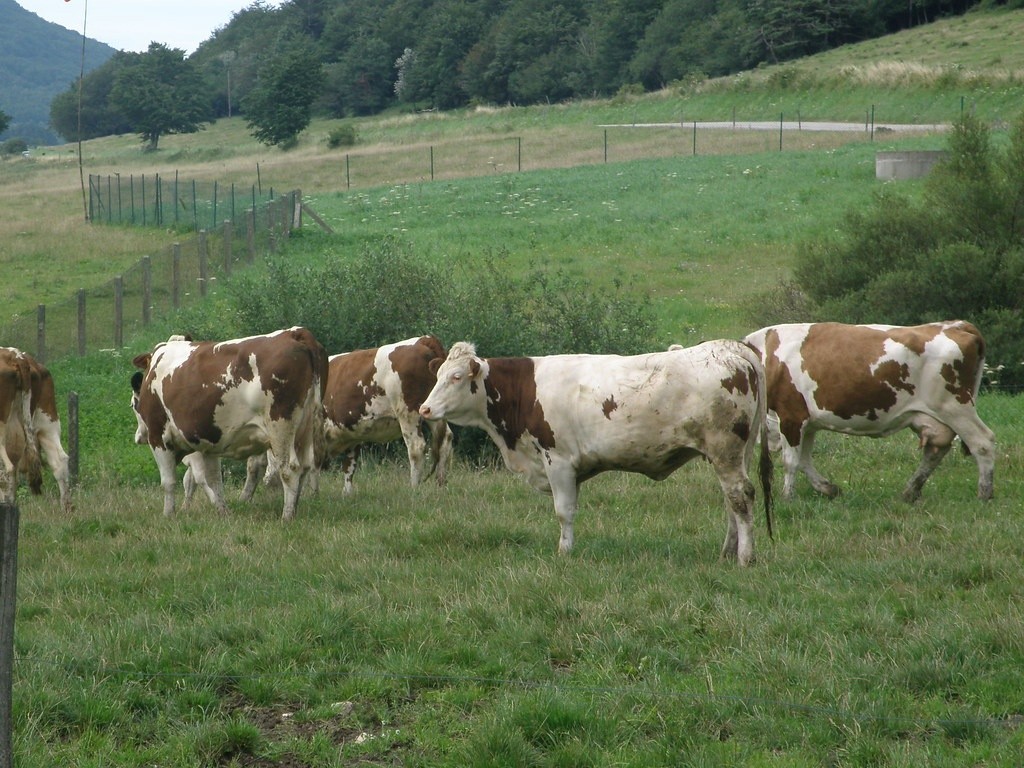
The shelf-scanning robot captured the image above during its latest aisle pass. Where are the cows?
[0,317,999,570]
